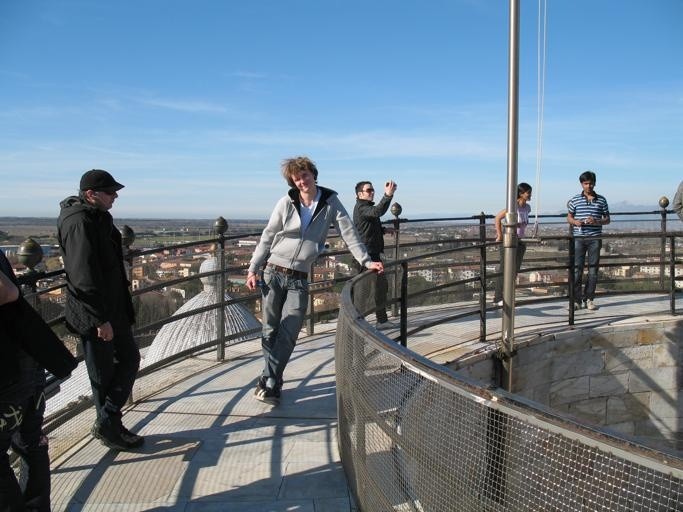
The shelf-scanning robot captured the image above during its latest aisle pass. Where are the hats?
[79,168,125,192]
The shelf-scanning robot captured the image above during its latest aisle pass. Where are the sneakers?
[92,420,131,452]
[375,322,395,331]
[253,371,284,407]
[585,299,597,311]
[574,302,581,310]
[114,425,146,449]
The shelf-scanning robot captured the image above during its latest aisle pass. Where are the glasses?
[103,191,118,197]
[357,188,375,193]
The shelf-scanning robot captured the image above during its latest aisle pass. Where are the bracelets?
[246,272,256,282]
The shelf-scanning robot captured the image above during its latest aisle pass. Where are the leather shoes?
[265,261,309,280]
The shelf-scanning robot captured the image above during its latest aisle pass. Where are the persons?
[352,179,398,331]
[494,182,533,307]
[567,170,611,311]
[245,155,385,407]
[55,168,145,452]
[0,247,79,512]
[672,179,683,223]
